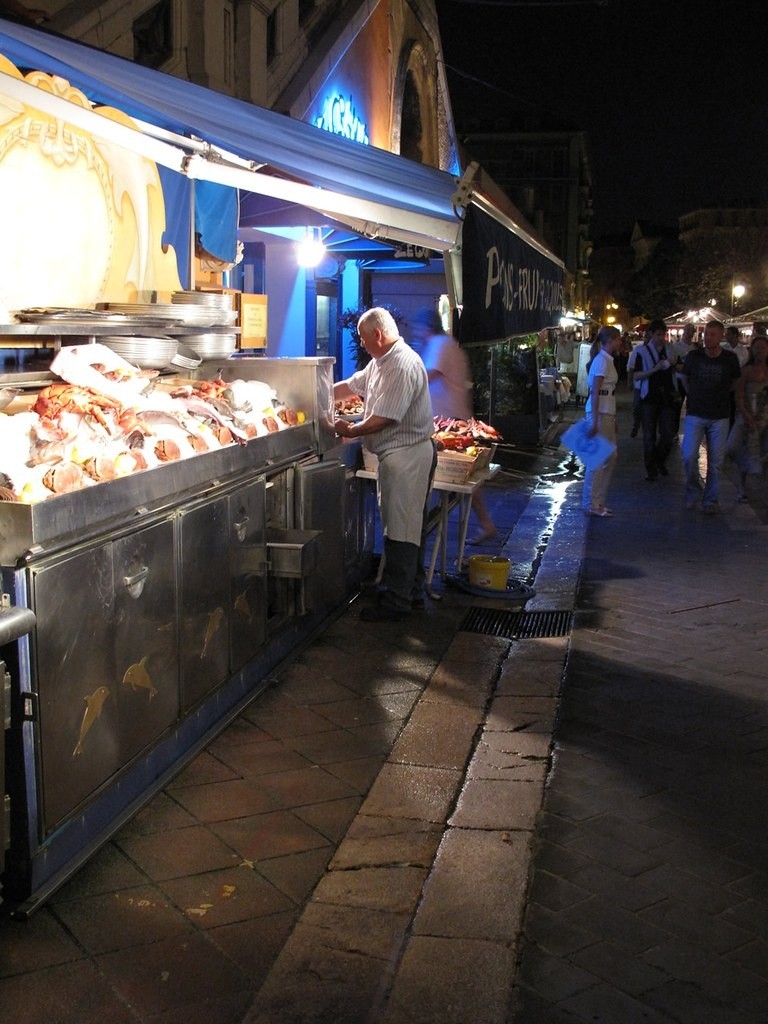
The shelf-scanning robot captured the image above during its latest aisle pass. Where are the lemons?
[439,433,455,438]
[466,446,478,457]
[113,455,137,474]
[296,412,305,423]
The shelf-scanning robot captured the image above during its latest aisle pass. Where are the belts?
[590,389,614,396]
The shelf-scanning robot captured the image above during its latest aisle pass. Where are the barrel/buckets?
[470,554,511,592]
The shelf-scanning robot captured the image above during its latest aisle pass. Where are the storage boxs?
[361,441,497,483]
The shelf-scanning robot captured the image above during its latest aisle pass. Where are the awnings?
[0,20,568,344]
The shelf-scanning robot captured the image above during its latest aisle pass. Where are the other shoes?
[359,603,412,623]
[630,426,638,437]
[703,500,719,514]
[655,460,668,477]
[377,588,425,609]
[640,466,655,481]
[737,488,749,502]
[465,530,497,546]
[685,494,698,509]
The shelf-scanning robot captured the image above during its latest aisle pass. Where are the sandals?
[586,504,612,513]
[586,507,614,518]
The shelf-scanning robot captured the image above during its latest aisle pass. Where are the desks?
[355,462,501,599]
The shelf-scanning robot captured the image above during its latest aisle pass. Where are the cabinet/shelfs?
[1,458,349,835]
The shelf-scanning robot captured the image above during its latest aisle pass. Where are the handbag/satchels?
[726,418,745,454]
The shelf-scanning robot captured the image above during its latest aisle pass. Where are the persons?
[333,307,437,620]
[582,326,623,518]
[681,321,743,514]
[410,308,497,545]
[727,336,768,503]
[613,318,749,480]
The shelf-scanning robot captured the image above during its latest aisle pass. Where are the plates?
[14,290,239,371]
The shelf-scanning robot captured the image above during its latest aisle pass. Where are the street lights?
[731,276,745,319]
[606,296,618,326]
